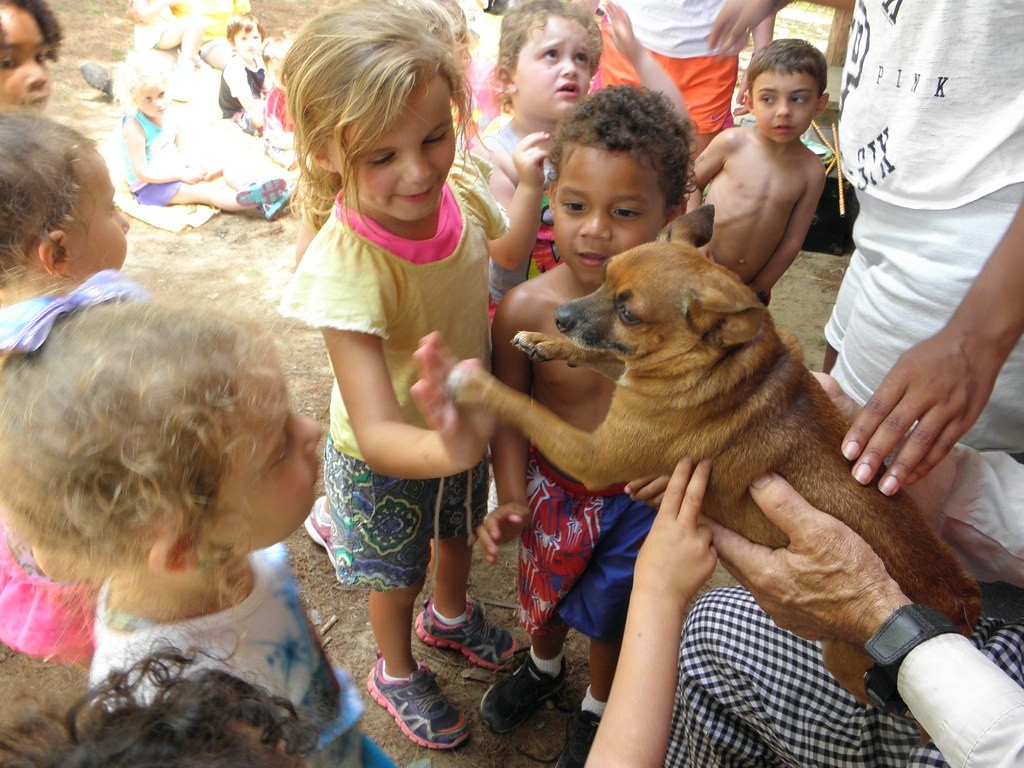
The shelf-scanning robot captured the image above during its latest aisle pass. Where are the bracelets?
[865,603,961,723]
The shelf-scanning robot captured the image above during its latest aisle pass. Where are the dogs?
[450,203,981,746]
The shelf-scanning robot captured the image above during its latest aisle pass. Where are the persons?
[79,0,295,221]
[0,648,320,767]
[480,0,1024,768]
[0,271,398,767]
[0,0,131,662]
[261,0,517,750]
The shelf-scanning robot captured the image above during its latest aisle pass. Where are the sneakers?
[80,61,114,97]
[366,650,469,750]
[555,701,602,768]
[481,650,566,732]
[303,495,338,569]
[235,175,298,220]
[414,596,523,671]
[171,61,197,105]
